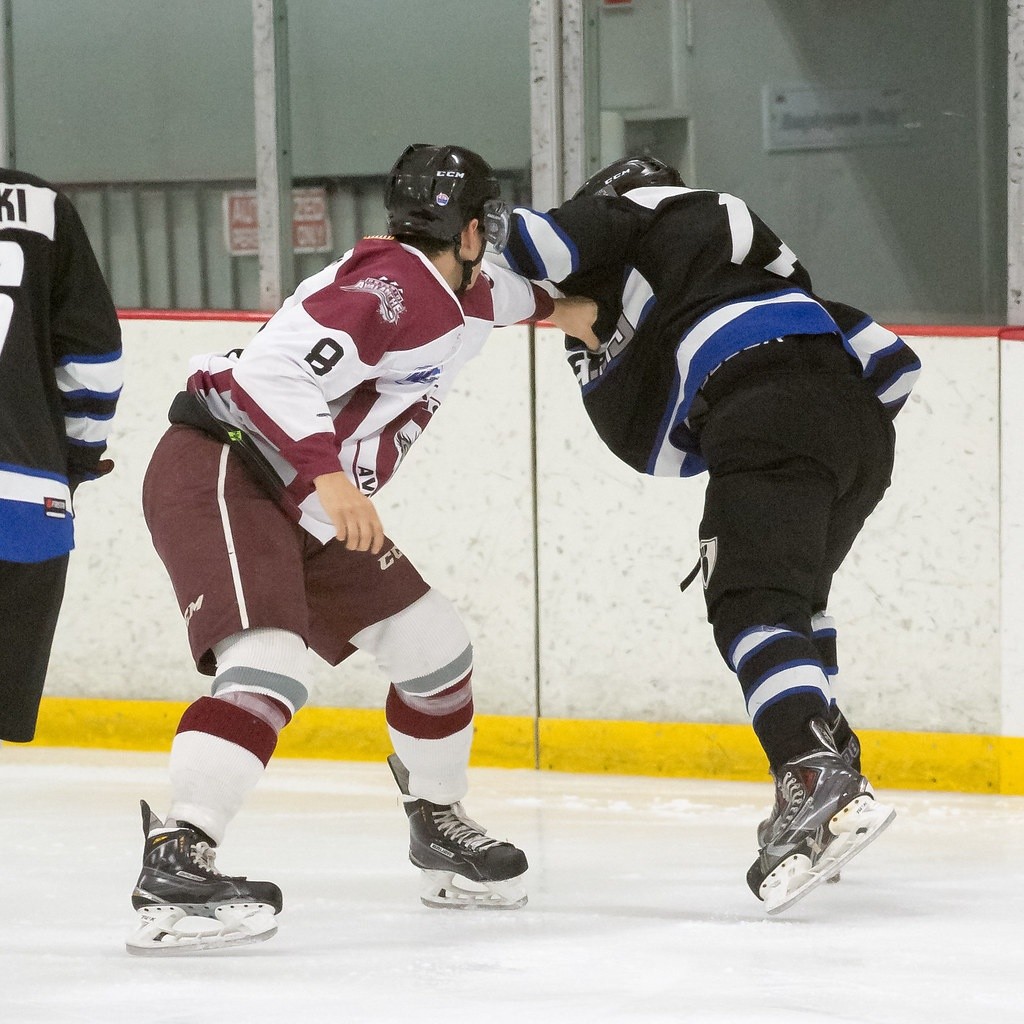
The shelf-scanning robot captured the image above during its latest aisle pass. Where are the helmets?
[384,143,502,243]
[571,156,686,200]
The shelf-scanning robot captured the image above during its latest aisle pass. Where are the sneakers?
[386,753,528,910]
[746,698,897,916]
[126,800,283,949]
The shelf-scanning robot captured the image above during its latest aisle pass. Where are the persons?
[124,143,600,956]
[0,168,124,743]
[505,151,922,914]
